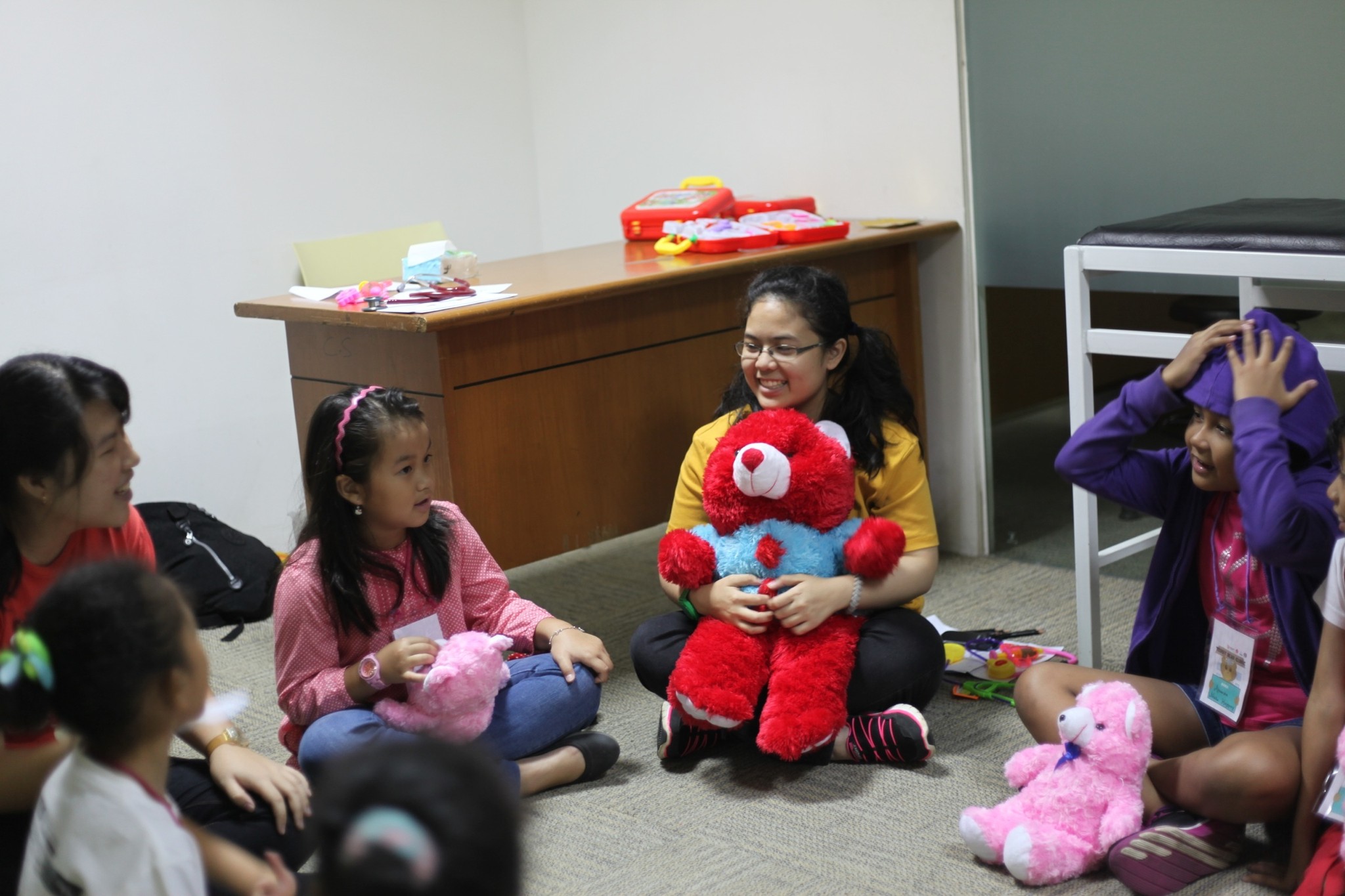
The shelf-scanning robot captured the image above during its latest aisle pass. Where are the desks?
[1061,194,1345,675]
[227,206,963,585]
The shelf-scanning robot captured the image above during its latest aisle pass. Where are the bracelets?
[546,622,588,650]
[840,572,864,615]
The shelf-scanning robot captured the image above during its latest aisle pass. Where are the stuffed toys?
[365,628,516,743]
[656,405,912,763]
[957,673,1156,889]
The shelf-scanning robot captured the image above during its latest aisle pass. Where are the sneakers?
[1107,811,1245,896]
[844,703,936,763]
[657,700,753,760]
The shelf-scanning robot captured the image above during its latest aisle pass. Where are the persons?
[272,381,622,809]
[1240,407,1345,896]
[1014,303,1344,888]
[628,261,947,769]
[0,353,315,896]
[1,556,303,896]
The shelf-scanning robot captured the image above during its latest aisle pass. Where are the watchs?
[201,721,249,757]
[360,651,389,692]
[675,588,700,622]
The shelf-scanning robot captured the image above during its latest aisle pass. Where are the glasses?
[730,340,826,361]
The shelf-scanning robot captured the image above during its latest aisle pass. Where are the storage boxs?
[618,191,849,260]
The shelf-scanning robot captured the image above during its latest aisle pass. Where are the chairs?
[294,221,456,296]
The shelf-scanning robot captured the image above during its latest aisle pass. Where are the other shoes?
[521,730,620,792]
